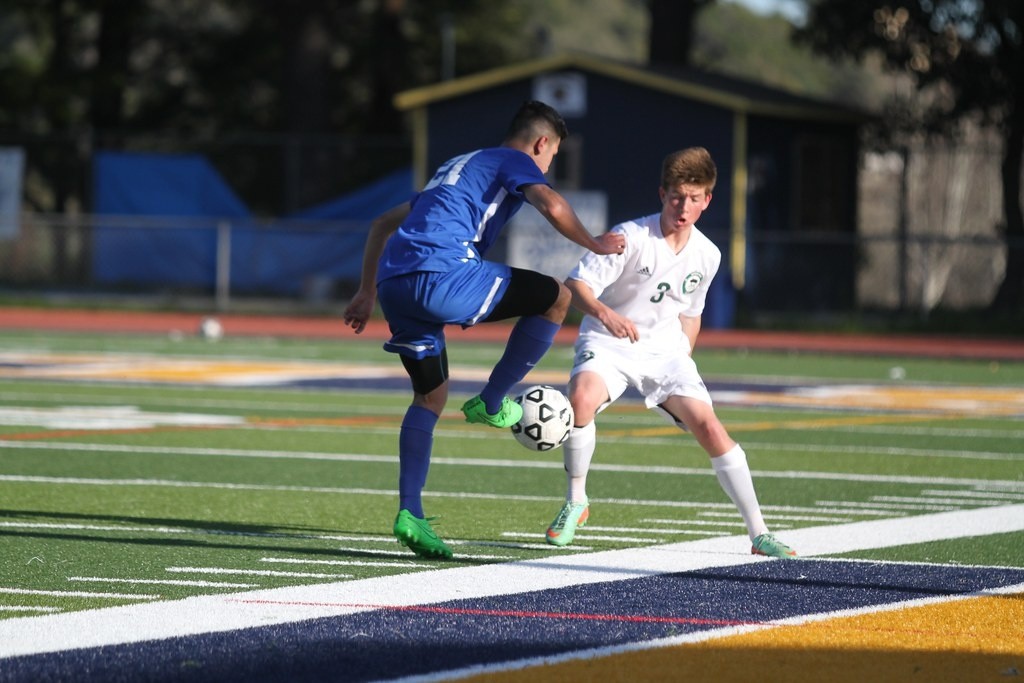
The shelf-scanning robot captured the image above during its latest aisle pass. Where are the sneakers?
[751,534,796,558]
[460,394,523,429]
[393,509,453,561]
[546,495,589,547]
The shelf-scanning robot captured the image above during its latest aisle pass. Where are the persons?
[545,145,802,557]
[341,101,625,560]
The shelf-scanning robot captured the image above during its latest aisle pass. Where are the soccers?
[509,385,575,452]
[200,318,224,339]
